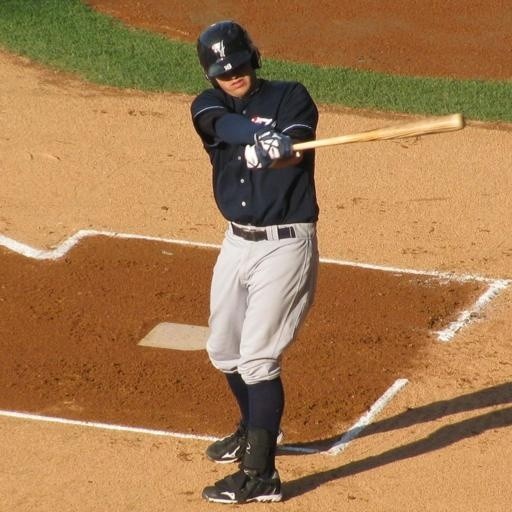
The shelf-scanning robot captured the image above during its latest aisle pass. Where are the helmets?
[197,21,262,88]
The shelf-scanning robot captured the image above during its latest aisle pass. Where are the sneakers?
[202,421,283,504]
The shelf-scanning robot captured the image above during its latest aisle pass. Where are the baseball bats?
[243,111,466,161]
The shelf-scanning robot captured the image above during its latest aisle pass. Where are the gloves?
[254,127,291,168]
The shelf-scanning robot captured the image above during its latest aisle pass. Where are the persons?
[190,21,319,503]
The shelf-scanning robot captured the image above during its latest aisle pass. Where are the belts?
[231,222,295,242]
